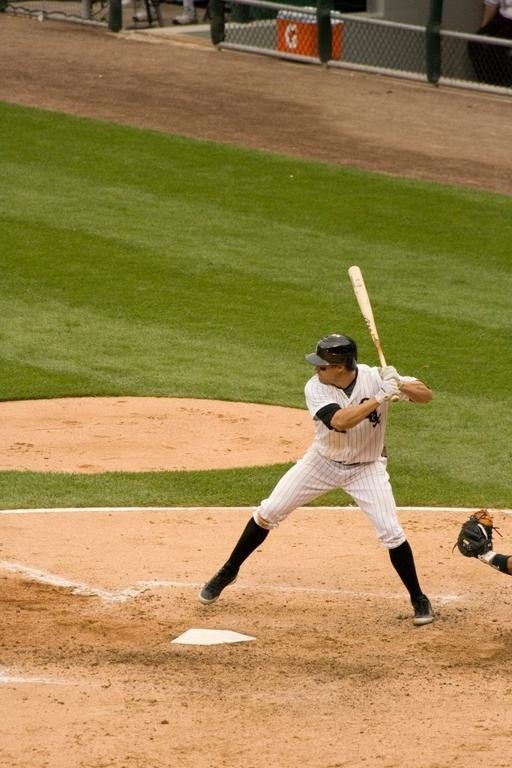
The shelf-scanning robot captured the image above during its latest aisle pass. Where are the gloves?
[375,365,402,405]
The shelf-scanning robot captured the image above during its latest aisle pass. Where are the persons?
[458,511,511,573]
[466,0,512,87]
[133,1,197,25]
[199,334,434,625]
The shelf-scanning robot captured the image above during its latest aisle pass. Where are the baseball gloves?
[457,508,494,557]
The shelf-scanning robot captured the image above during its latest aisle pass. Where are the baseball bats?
[348,266,400,402]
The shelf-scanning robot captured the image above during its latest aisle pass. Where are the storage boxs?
[273,9,345,64]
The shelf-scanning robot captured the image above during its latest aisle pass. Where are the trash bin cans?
[277,5,343,60]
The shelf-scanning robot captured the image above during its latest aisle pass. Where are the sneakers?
[199,567,237,605]
[133,10,159,21]
[173,13,196,25]
[409,595,433,625]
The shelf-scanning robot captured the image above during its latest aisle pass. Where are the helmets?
[305,335,358,371]
